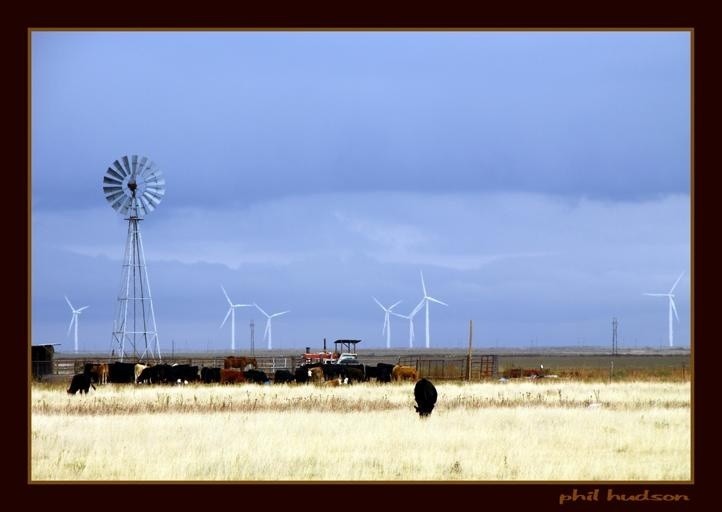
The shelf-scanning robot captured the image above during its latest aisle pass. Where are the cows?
[79,363,421,385]
[67,374,96,395]
[413,377,437,417]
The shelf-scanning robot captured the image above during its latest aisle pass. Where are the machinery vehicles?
[304,338,362,364]
